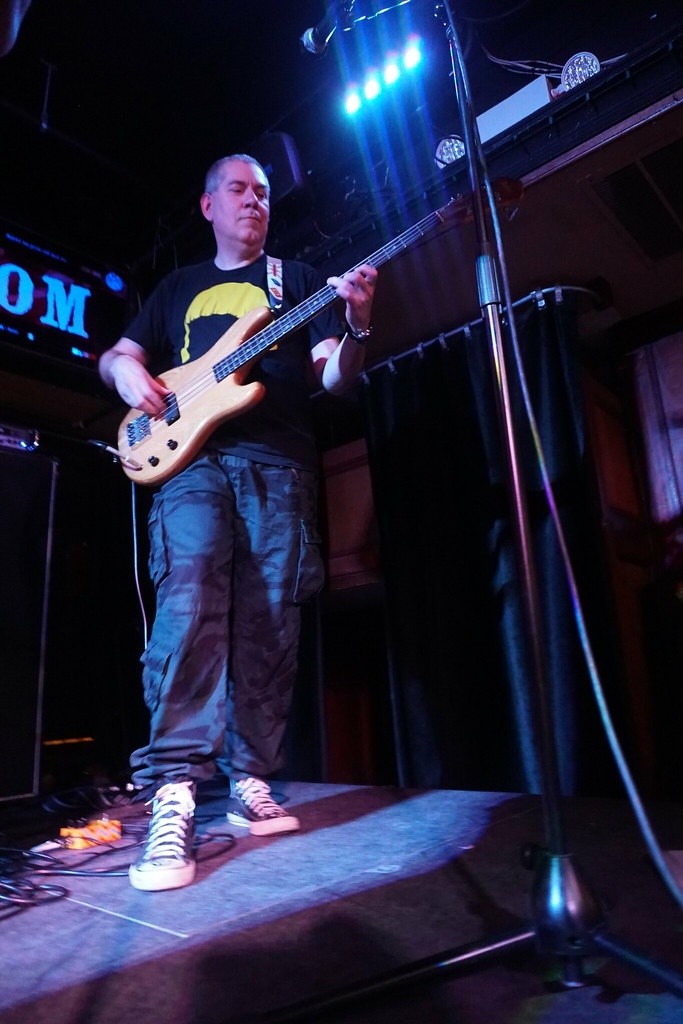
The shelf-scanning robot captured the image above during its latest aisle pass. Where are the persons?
[98,153,378,891]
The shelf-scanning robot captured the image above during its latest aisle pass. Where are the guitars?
[115,171,528,487]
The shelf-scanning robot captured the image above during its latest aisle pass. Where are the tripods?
[261,0,683,1023]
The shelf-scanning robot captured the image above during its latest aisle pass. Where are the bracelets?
[344,321,375,342]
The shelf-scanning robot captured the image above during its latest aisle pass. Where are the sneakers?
[128,777,199,892]
[223,774,302,837]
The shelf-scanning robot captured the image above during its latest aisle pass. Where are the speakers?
[0,442,59,803]
